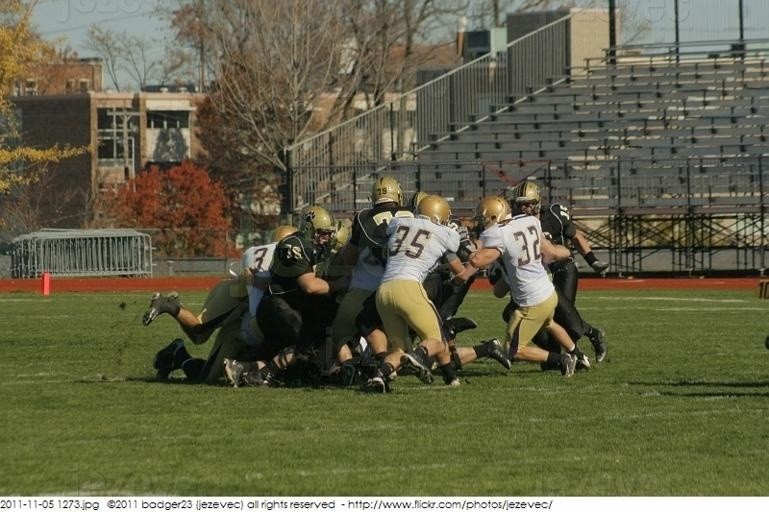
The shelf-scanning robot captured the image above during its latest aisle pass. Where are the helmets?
[510,182,542,215]
[412,191,453,227]
[474,197,512,233]
[300,206,352,251]
[271,225,299,243]
[372,176,404,208]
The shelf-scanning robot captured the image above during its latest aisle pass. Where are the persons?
[136,173,606,393]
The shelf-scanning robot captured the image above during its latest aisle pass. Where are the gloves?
[592,261,610,278]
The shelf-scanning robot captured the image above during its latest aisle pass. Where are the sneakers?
[153,339,184,379]
[485,338,511,370]
[327,352,461,394]
[143,291,181,326]
[225,357,302,388]
[442,316,478,339]
[557,330,606,377]
